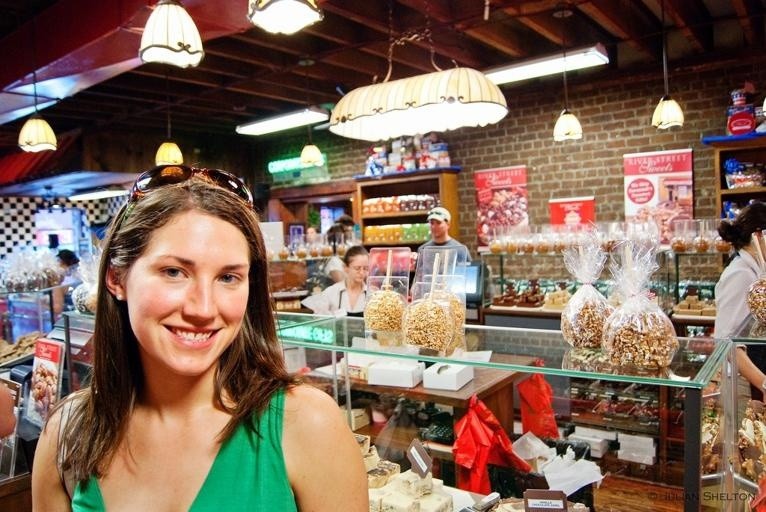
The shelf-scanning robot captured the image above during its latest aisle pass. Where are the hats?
[56,250,79,265]
[427,207,450,222]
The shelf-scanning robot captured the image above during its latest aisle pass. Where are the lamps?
[454,40,610,86]
[246,0,324,37]
[155,72,183,166]
[301,39,324,169]
[553,9,583,142]
[235,60,329,137]
[17,72,58,154]
[651,0,684,129]
[138,0,205,70]
[329,0,510,145]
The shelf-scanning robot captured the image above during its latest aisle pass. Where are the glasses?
[128,165,255,208]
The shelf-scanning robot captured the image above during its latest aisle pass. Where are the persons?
[30,165,369,512]
[55,248,80,282]
[712,201,765,404]
[303,223,321,243]
[326,225,346,244]
[333,214,355,241]
[313,246,376,316]
[414,206,474,264]
